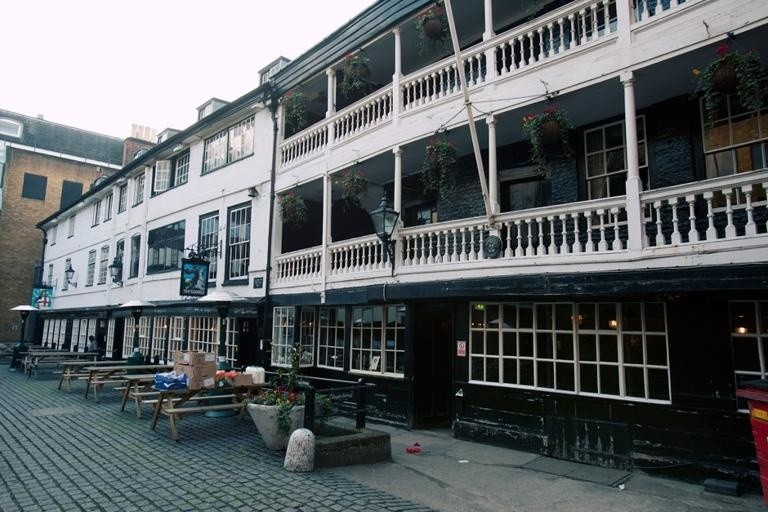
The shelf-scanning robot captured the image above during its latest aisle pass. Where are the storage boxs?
[172,350,216,365]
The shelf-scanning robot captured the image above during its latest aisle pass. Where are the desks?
[19,349,101,379]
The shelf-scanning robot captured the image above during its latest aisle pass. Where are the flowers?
[416,5,448,41]
[421,139,460,198]
[280,51,369,135]
[687,44,766,129]
[278,166,367,227]
[519,103,573,177]
[243,347,330,436]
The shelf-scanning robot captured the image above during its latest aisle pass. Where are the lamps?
[64,264,77,288]
[107,257,122,283]
[369,198,399,277]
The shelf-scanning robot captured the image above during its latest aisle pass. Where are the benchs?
[14,358,268,443]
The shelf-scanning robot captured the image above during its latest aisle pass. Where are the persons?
[89,336,98,350]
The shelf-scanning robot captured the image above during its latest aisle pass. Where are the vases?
[430,152,439,167]
[713,62,737,95]
[247,401,304,451]
[542,119,558,138]
[422,17,441,38]
